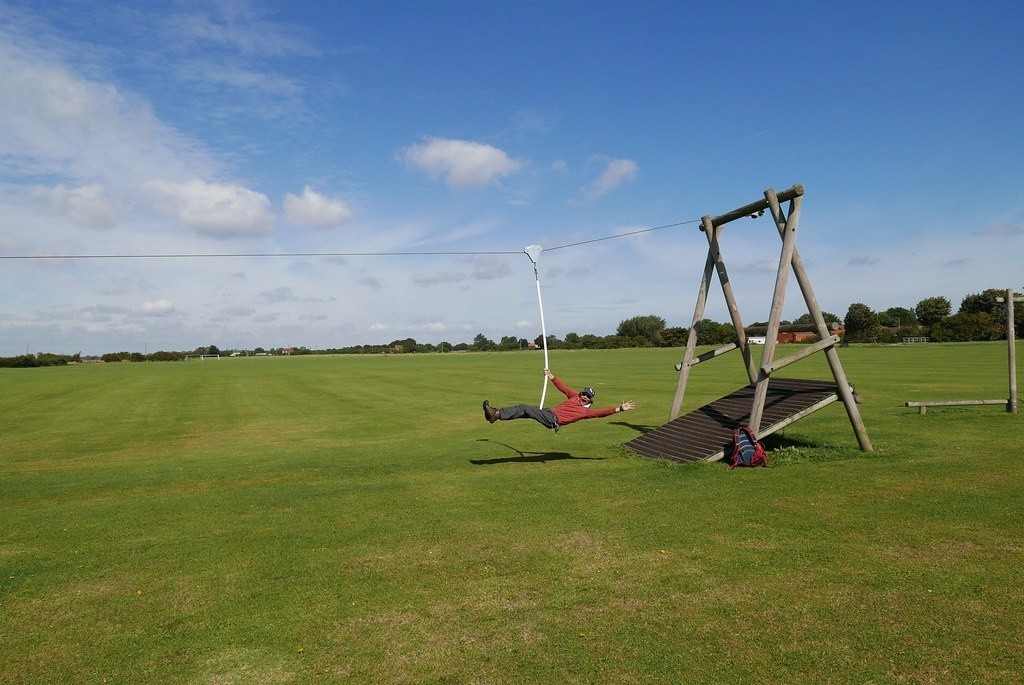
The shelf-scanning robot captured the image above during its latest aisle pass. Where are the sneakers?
[482,399,501,424]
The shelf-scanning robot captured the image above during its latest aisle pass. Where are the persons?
[483,368,635,429]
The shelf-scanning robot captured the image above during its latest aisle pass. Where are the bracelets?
[620,407,622,411]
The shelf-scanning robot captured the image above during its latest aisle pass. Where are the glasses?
[581,392,591,399]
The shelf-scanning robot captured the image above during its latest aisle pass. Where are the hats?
[581,386,596,398]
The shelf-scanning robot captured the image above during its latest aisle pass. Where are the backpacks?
[727,424,768,470]
